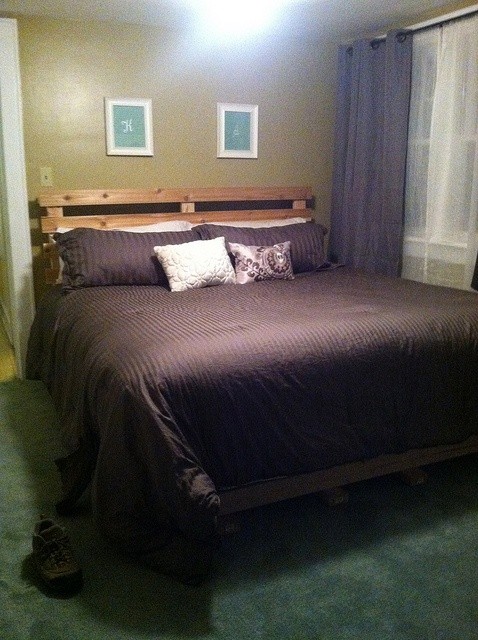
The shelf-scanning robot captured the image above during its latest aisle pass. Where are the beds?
[36,183,478,516]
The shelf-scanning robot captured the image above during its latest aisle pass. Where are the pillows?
[154,235,236,293]
[53,228,201,287]
[229,241,295,284]
[193,223,331,270]
[54,221,192,285]
[200,218,306,228]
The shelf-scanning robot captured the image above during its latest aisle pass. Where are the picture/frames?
[104,97,154,157]
[216,103,258,159]
